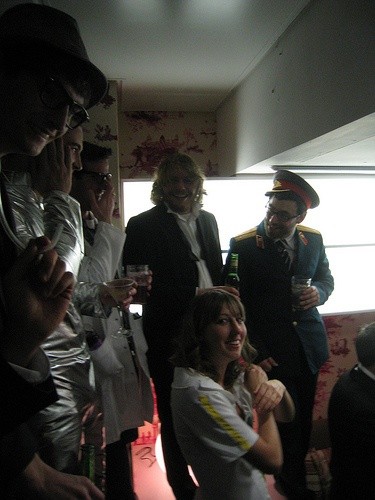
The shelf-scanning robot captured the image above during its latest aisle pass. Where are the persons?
[121,151,375,500]
[0,0,154,500]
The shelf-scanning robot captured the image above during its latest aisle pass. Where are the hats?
[265,170,319,210]
[0,3,108,109]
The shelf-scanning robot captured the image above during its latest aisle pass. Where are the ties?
[276,239,294,271]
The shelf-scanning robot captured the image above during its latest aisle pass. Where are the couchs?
[301,309,375,500]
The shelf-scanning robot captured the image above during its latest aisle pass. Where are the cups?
[126,265,150,305]
[290,276,312,312]
[104,278,134,305]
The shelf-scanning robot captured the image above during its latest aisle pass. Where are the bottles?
[225,253,240,292]
[80,443,102,487]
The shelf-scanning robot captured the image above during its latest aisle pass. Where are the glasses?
[266,201,298,222]
[22,62,89,130]
[80,170,112,184]
